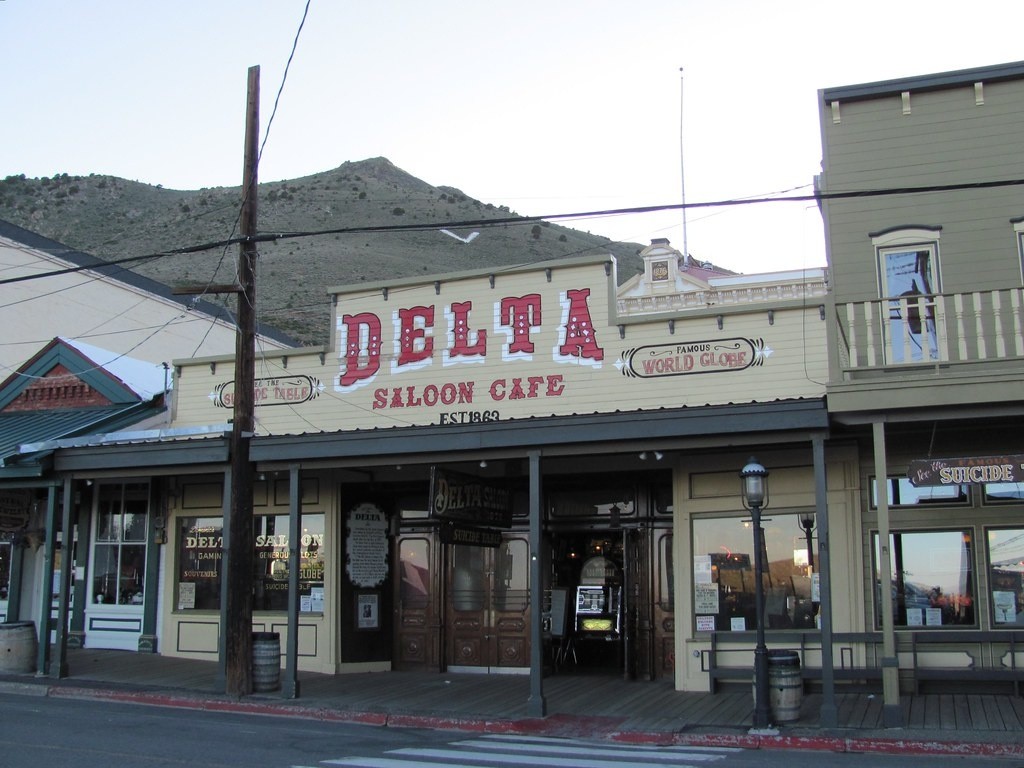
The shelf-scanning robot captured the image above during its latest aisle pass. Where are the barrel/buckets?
[251,631,282,692]
[0,621,38,672]
[752,649,803,721]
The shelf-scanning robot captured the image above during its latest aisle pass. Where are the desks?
[93,574,141,605]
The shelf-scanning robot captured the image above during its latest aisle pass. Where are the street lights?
[797,513,817,573]
[739,462,770,729]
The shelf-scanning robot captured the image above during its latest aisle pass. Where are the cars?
[877,579,932,623]
